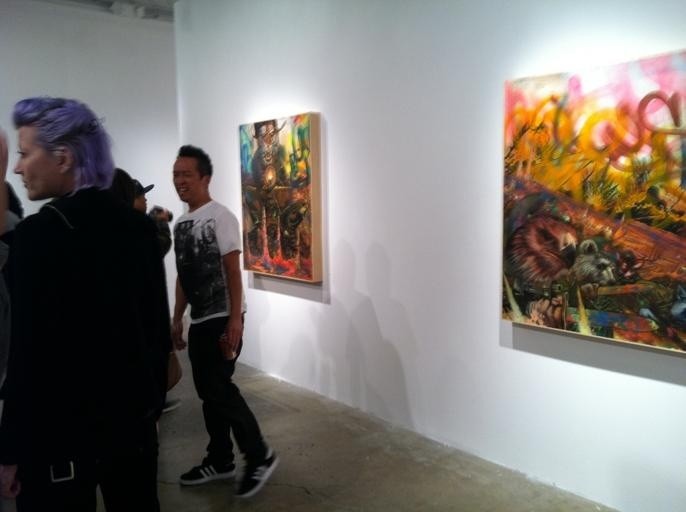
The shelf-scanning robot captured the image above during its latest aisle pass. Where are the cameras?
[150,205,173,221]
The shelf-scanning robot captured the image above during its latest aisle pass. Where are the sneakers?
[178,458,236,486]
[235,446,281,499]
[162,399,180,413]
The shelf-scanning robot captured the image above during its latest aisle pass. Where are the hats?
[132,179,155,200]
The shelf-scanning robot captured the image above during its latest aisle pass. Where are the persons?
[171,145,281,500]
[0,94,183,511]
